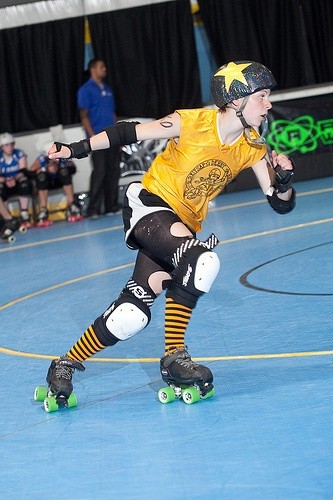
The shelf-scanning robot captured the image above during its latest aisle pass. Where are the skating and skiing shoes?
[33,356,85,412]
[18,212,34,233]
[0,216,28,244]
[37,208,53,227]
[64,204,84,222]
[158,351,216,404]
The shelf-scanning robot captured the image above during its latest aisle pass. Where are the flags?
[189,1,218,105]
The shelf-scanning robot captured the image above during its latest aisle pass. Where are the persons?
[27,151,83,227]
[78,58,128,220]
[0,198,27,243]
[34,61,298,413]
[0,132,36,245]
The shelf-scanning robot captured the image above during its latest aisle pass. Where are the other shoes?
[105,208,123,216]
[90,212,98,220]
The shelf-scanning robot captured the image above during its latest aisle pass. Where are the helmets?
[212,61,276,108]
[0,133,15,147]
[44,142,54,155]
